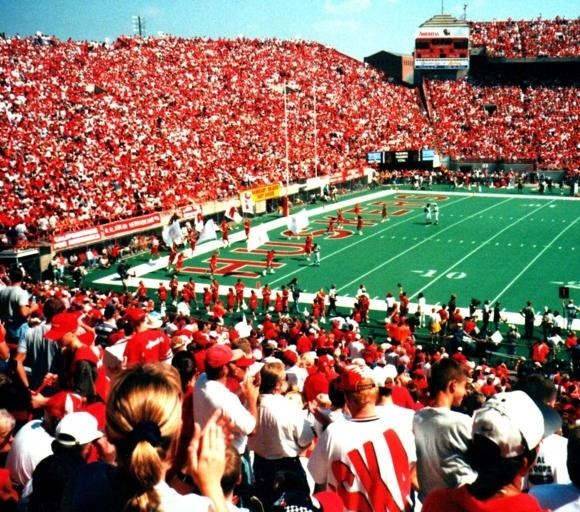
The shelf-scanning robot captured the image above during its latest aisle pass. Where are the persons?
[388,73,580,195]
[13,299,65,419]
[6,391,86,495]
[467,298,543,415]
[88,245,283,380]
[421,389,563,512]
[372,285,430,409]
[20,412,120,512]
[308,361,420,512]
[106,364,248,512]
[171,350,197,472]
[431,294,467,363]
[194,344,260,508]
[0,409,20,512]
[0,325,10,361]
[278,474,316,512]
[244,361,312,512]
[432,204,439,224]
[0,245,88,324]
[414,358,475,503]
[284,245,372,428]
[424,204,432,225]
[83,402,107,463]
[544,299,580,433]
[219,445,251,512]
[468,16,580,58]
[527,427,580,512]
[0,31,388,245]
[28,311,97,411]
[512,375,574,494]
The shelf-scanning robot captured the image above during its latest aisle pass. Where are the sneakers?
[261,270,268,277]
[169,267,173,273]
[270,270,275,274]
[173,274,178,279]
[210,274,214,280]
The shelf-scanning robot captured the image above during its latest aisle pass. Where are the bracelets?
[176,470,196,489]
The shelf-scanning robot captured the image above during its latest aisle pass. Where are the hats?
[70,345,98,374]
[316,353,337,371]
[40,311,81,342]
[470,389,564,460]
[412,366,426,378]
[234,352,266,376]
[507,323,518,331]
[336,363,379,391]
[121,307,148,328]
[203,342,245,368]
[54,410,105,448]
[42,390,85,417]
[119,327,174,372]
[281,349,299,366]
